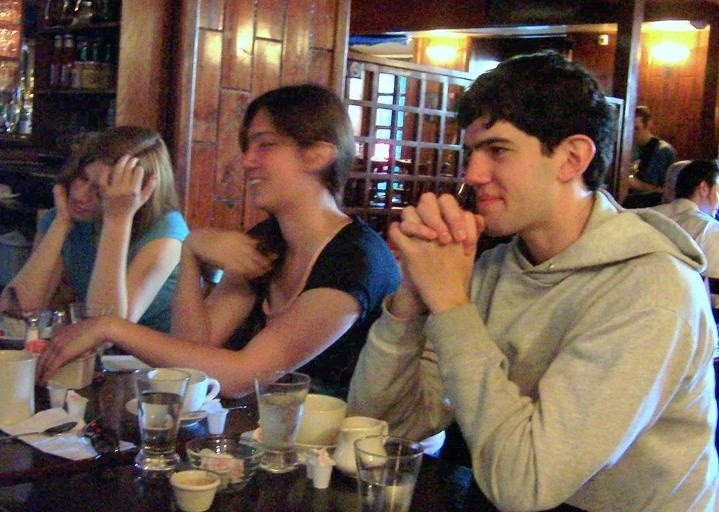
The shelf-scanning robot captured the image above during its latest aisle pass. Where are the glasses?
[81,412,122,459]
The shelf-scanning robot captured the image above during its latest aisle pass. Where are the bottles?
[19,70,35,137]
[47,31,119,89]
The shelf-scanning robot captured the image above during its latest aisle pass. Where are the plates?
[124,397,206,424]
[253,429,337,457]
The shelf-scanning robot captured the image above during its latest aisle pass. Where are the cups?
[129,368,192,472]
[352,435,423,512]
[207,408,229,435]
[296,390,346,441]
[0,301,114,429]
[147,366,222,411]
[4,102,20,133]
[253,369,310,473]
[335,416,391,481]
[169,469,220,512]
[306,450,337,489]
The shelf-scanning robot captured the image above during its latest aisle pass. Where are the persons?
[35,82,403,402]
[0,124,204,336]
[626,105,719,346]
[346,51,719,512]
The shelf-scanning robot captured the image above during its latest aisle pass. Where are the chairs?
[349,151,473,237]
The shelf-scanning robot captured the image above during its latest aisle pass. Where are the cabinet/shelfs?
[24,1,176,164]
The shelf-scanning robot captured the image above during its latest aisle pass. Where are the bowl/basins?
[185,435,266,492]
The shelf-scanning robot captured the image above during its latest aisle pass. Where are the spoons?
[1,421,78,444]
